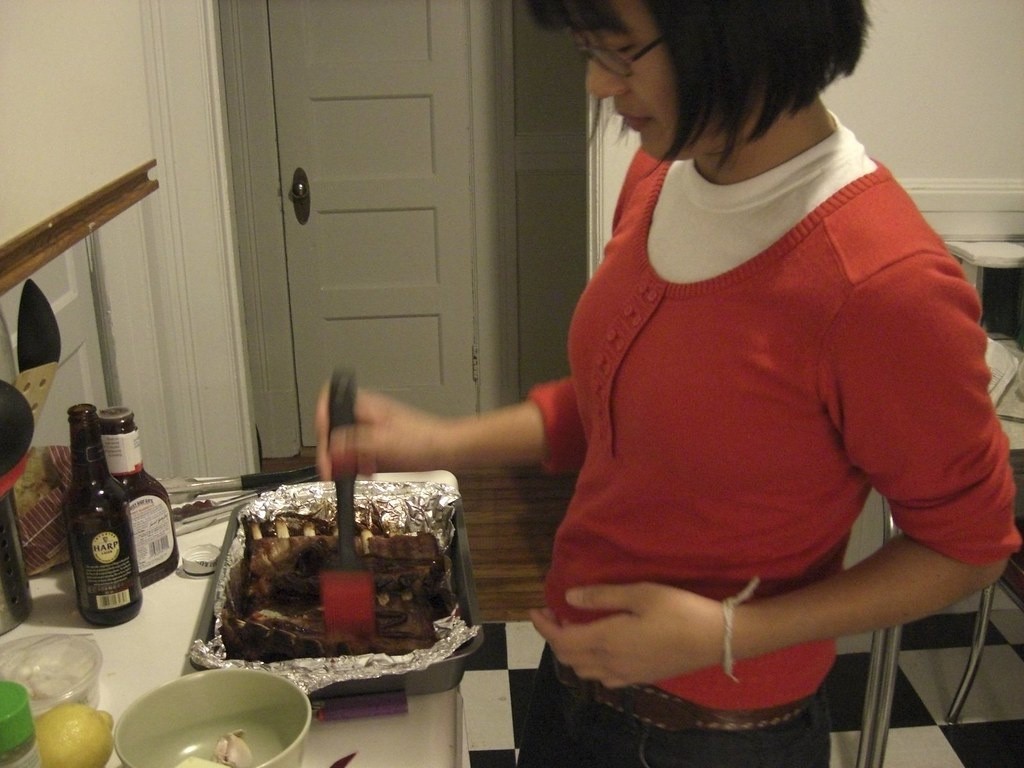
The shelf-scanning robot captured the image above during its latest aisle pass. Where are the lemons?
[31,702,113,768]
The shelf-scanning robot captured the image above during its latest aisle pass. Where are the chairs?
[836,237,1023,767]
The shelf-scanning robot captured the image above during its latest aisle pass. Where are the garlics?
[213,736,253,767]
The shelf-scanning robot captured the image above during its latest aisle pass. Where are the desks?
[0,503,464,767]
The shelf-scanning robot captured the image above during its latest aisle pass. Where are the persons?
[316,0,1020,768]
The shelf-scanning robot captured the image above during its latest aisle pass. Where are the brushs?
[318,367,378,641]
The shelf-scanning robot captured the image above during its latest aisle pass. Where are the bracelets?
[722,574,759,682]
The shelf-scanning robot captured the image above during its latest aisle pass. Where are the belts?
[552,655,818,731]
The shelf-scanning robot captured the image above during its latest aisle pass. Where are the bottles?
[96,406,180,590]
[0,679,43,768]
[60,403,143,627]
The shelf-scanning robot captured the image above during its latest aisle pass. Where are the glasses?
[569,28,664,79]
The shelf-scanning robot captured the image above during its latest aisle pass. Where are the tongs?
[155,465,319,536]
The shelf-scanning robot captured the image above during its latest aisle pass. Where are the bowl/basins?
[0,632,104,723]
[114,667,313,768]
